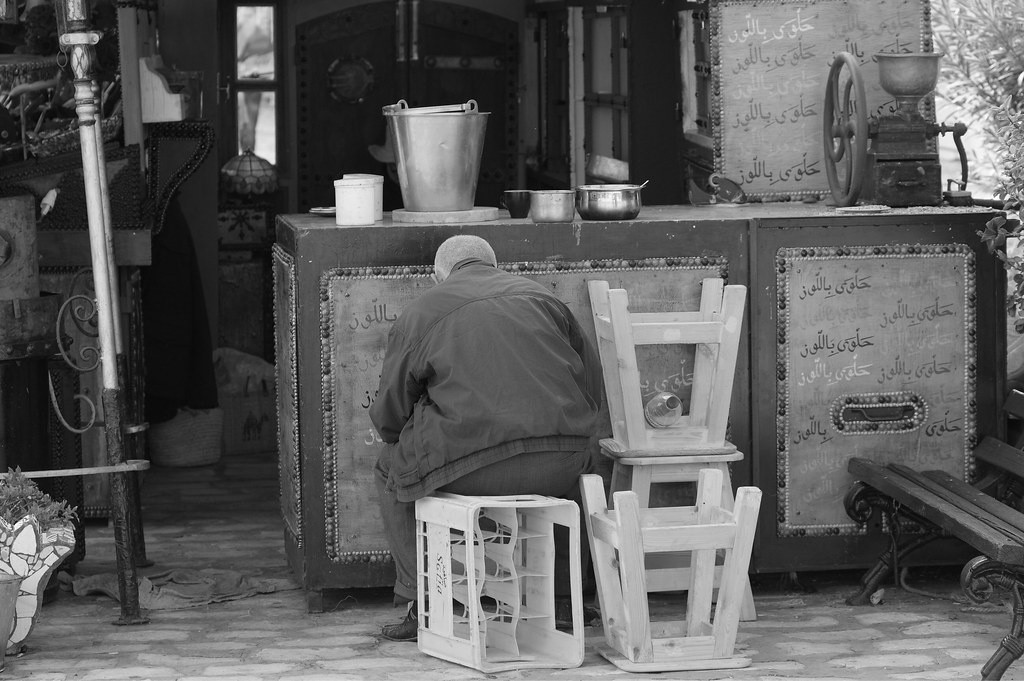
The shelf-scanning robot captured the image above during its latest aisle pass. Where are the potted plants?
[0,465,79,655]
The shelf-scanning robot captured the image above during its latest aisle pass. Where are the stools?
[587,277,747,457]
[594,448,757,621]
[414,493,586,672]
[580,473,762,673]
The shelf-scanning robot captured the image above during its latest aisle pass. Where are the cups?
[499,191,531,218]
[343,173,383,221]
[333,179,375,227]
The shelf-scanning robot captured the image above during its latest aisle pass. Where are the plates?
[309,207,336,216]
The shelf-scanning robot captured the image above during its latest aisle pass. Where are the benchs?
[843,388,1024,681]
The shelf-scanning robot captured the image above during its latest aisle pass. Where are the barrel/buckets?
[383,99,491,211]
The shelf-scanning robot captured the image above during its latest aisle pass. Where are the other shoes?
[382,608,427,641]
[554,601,600,628]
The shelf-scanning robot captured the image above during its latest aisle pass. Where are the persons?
[369,235,602,641]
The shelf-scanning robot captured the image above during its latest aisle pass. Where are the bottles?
[644,391,682,429]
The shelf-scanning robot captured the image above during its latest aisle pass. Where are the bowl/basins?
[529,190,575,223]
[574,184,646,220]
